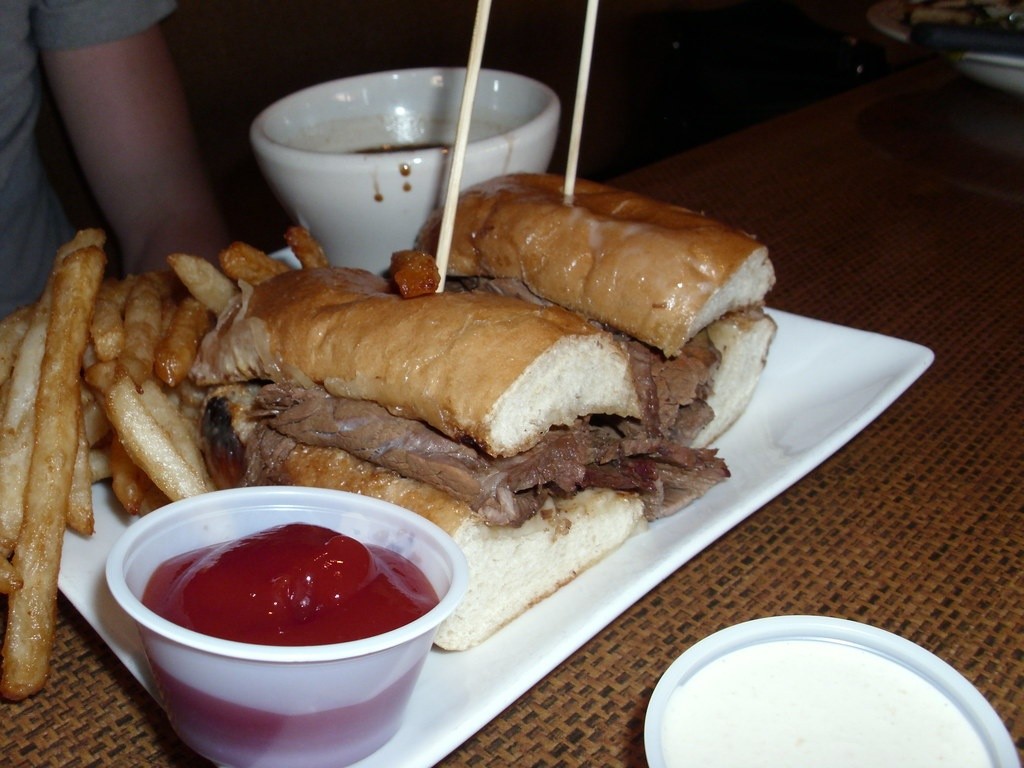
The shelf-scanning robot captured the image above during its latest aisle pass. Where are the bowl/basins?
[250,67,562,279]
[104,485,468,768]
[644,616,1021,768]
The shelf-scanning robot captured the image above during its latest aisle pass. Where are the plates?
[867,0,1024,95]
[54,307,940,768]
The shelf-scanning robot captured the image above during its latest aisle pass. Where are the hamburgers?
[187,174,780,650]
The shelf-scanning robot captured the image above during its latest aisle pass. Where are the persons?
[1,0,228,330]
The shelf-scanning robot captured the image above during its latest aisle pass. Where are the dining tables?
[0,57,1024,768]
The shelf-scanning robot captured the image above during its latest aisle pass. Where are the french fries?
[0,225,324,704]
